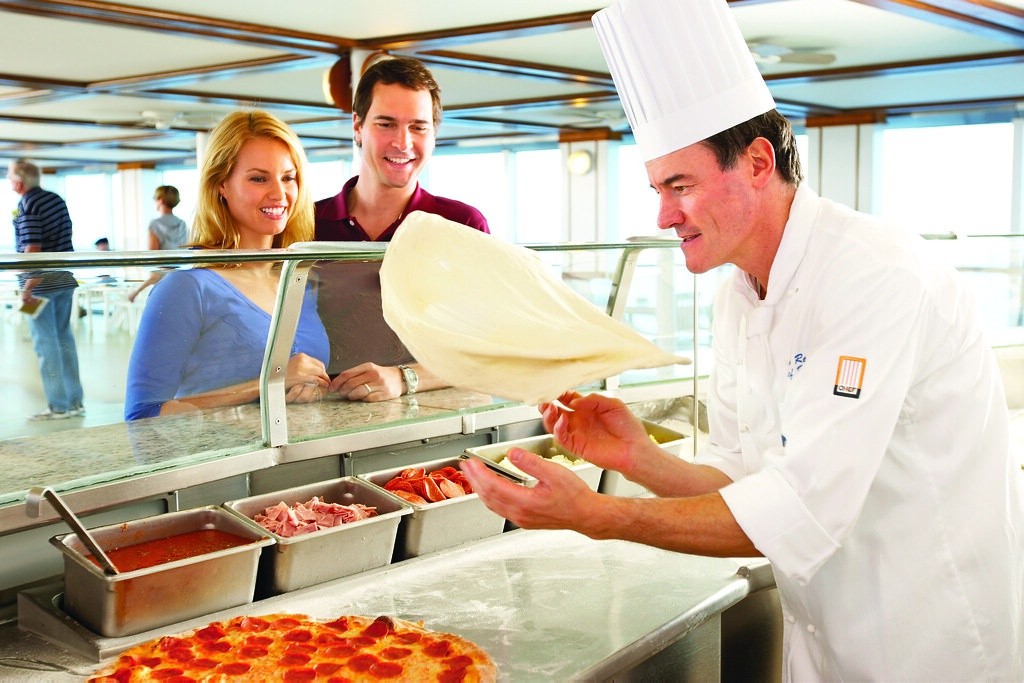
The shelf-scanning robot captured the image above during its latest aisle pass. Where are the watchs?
[395,364,418,395]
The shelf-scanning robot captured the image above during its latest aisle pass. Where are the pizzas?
[87,613,498,683]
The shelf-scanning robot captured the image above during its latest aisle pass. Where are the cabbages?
[498,434,661,473]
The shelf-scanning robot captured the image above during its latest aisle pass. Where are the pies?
[380,209,694,401]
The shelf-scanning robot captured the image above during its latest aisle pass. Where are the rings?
[312,392,318,396]
[364,383,372,393]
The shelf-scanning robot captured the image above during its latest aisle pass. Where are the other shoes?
[28,407,84,419]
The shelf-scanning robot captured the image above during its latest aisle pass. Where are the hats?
[591,0,776,162]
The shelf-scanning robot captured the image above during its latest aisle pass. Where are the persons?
[272,57,490,402]
[127,186,188,302]
[94,238,113,277]
[123,104,330,421]
[460,0,1023,683]
[6,163,86,421]
